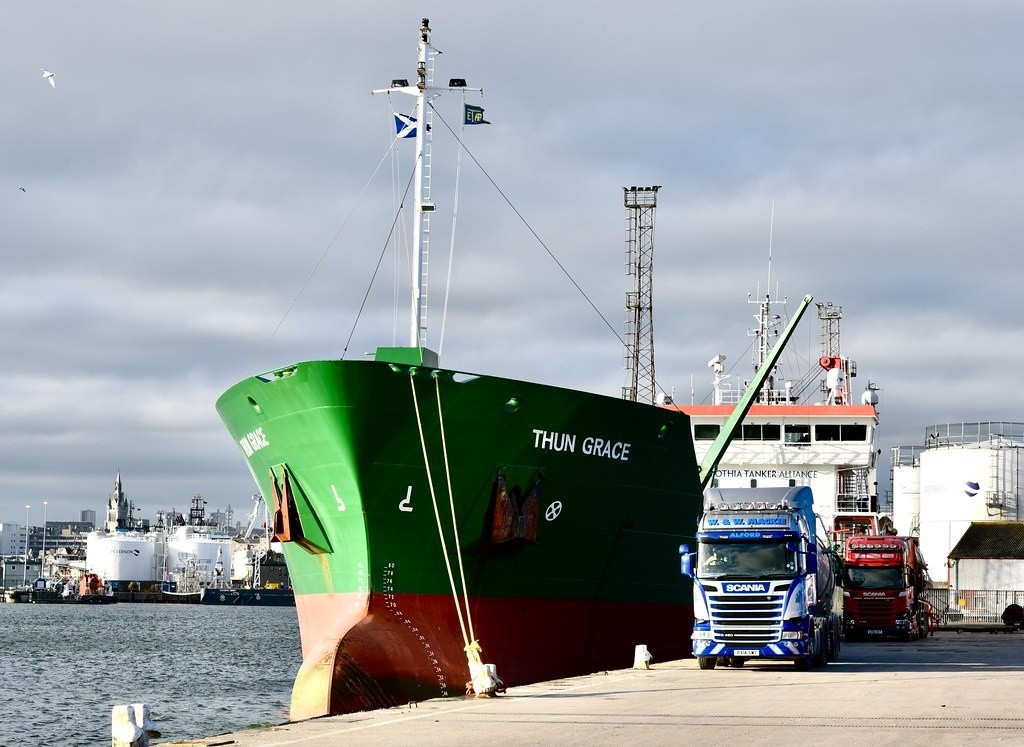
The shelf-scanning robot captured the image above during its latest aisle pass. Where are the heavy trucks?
[678,488,840,670]
[844,536,930,641]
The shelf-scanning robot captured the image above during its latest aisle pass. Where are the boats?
[211,15,880,727]
[201,546,295,606]
[4,571,119,605]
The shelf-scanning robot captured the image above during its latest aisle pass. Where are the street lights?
[41,502,48,580]
[23,505,31,589]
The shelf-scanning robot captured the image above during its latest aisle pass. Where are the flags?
[464,104,492,125]
[393,113,431,139]
